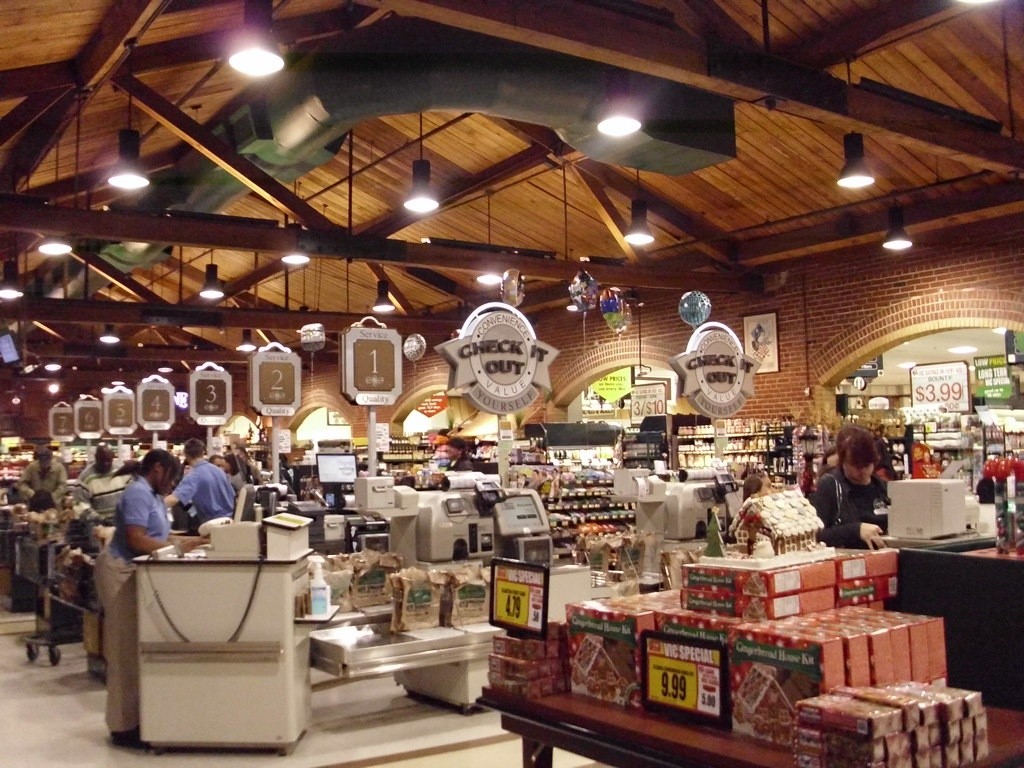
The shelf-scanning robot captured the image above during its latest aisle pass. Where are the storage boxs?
[487,549,990,768]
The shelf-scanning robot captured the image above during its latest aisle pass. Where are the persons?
[18,448,67,505]
[743,473,771,502]
[446,438,474,472]
[268,452,293,490]
[814,428,899,551]
[76,438,263,749]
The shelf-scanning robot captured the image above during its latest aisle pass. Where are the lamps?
[0,230,24,299]
[227,0,285,76]
[236,330,257,353]
[373,261,395,313]
[99,281,120,345]
[405,111,439,212]
[623,170,654,246]
[108,38,150,190]
[597,67,642,136]
[882,198,913,251]
[199,248,224,300]
[836,54,876,189]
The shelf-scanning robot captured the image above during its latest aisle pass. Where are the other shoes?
[110,727,149,750]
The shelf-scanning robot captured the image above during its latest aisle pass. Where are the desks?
[475,686,1024,768]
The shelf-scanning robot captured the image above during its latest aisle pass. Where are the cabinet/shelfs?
[378,431,795,556]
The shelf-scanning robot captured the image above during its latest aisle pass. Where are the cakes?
[699,489,835,568]
